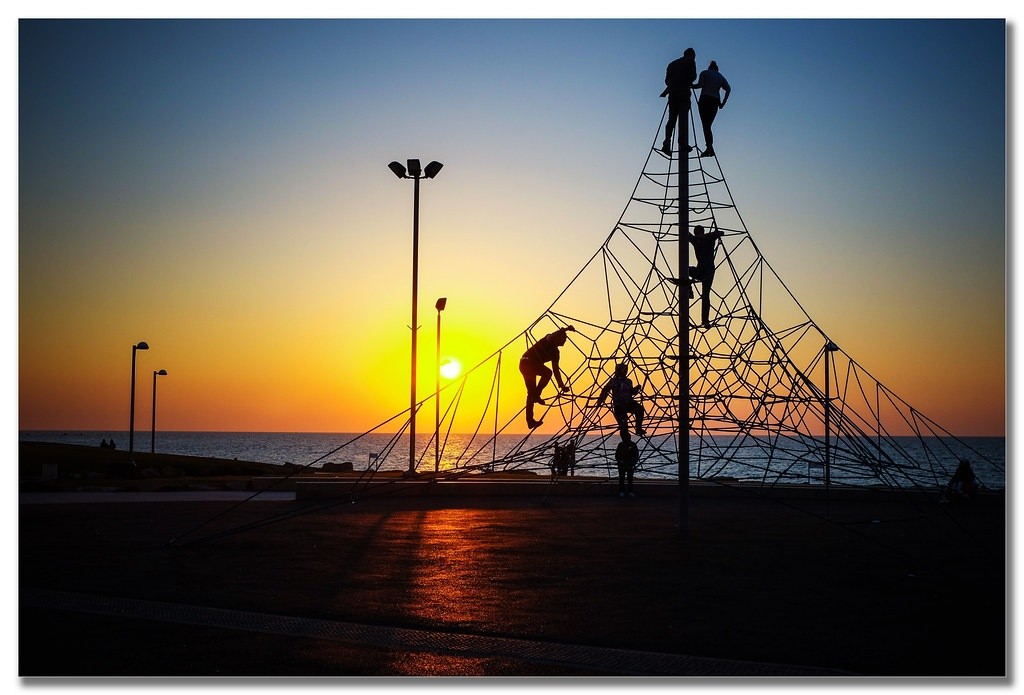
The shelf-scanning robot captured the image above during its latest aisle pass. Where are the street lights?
[387,158,444,475]
[434,296,446,469]
[130,342,149,451]
[152,370,167,454]
[823,342,838,488]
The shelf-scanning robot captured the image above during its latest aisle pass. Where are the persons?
[661,48,697,155]
[691,61,731,156]
[519,325,575,429]
[596,364,646,446]
[555,439,577,480]
[941,458,980,506]
[615,434,639,498]
[100,439,116,450]
[688,225,724,329]
